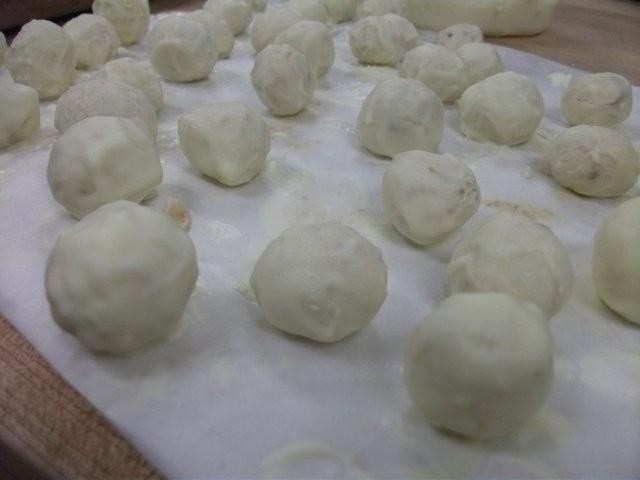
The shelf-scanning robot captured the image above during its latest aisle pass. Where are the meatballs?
[0,0,640,441]
